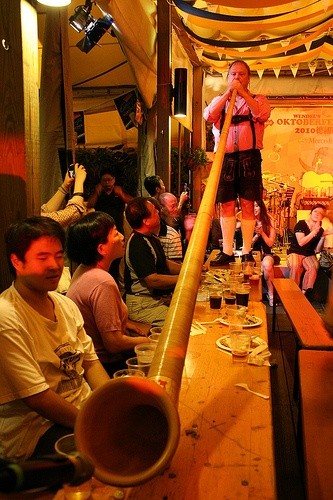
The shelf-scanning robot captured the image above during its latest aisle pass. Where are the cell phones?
[184,181,187,191]
[68,163,77,179]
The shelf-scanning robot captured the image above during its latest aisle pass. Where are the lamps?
[66,0,113,53]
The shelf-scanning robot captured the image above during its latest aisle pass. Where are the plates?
[218,315,263,328]
[215,334,267,352]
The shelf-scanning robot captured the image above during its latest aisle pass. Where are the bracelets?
[58,187,66,194]
[72,192,84,197]
[61,185,69,194]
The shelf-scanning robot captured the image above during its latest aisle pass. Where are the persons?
[0,163,333,500]
[204,60,271,267]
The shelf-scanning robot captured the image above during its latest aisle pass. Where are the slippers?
[267,294,277,306]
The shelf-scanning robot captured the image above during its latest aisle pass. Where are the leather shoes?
[242,251,256,266]
[210,251,236,266]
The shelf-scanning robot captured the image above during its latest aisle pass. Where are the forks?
[235,383,270,399]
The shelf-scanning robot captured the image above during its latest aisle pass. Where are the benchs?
[271,278,333,500]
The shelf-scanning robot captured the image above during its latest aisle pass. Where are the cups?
[197,261,260,367]
[113,327,164,378]
[54,434,92,500]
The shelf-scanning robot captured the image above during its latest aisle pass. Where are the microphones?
[0,451,95,492]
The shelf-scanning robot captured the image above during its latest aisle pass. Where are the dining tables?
[193,249,263,301]
[89,301,274,500]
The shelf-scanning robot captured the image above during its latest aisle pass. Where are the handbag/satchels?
[320,250,333,268]
